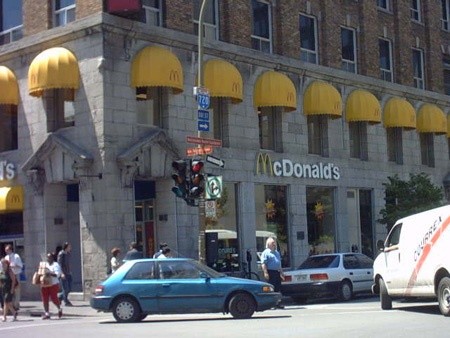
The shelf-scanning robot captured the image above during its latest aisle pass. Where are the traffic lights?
[189,159,204,198]
[171,159,186,198]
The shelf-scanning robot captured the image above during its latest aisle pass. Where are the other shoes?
[58,309,62,318]
[276,305,285,309]
[42,315,50,320]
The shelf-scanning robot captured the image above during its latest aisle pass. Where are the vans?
[371,204,450,316]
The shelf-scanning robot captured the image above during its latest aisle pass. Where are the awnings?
[253,70,297,112]
[345,89,382,125]
[130,45,184,96]
[303,80,343,120]
[447,113,450,138]
[196,59,244,104]
[383,97,417,131]
[0,65,19,106]
[27,47,80,99]
[416,103,447,135]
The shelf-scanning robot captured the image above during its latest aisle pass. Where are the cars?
[90,258,282,323]
[281,253,374,304]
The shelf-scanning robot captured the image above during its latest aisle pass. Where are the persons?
[153,243,173,259]
[122,243,143,260]
[111,248,122,275]
[57,242,72,306]
[261,239,285,310]
[0,244,23,321]
[37,252,63,320]
